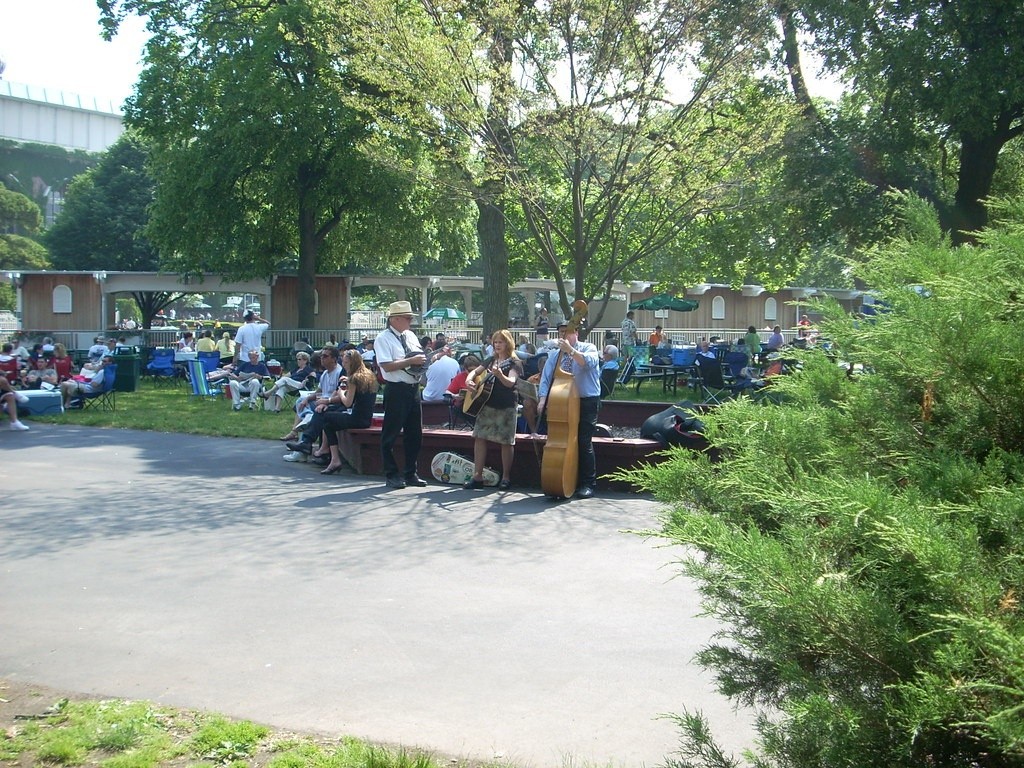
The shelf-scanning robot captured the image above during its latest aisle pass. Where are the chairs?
[443,394,475,431]
[0,349,316,413]
[600,338,836,405]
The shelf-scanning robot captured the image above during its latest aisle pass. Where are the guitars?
[463,357,514,417]
[405,342,461,375]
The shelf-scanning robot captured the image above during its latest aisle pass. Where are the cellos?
[542,300,589,498]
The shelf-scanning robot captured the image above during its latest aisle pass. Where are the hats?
[223,332,230,338]
[96,336,106,342]
[387,301,419,317]
[556,320,568,330]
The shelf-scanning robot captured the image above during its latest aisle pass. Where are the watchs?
[568,348,576,356]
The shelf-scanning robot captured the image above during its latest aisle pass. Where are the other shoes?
[282,452,308,462]
[286,442,312,455]
[463,479,484,489]
[499,480,511,489]
[577,486,595,499]
[295,414,313,432]
[312,458,330,465]
[256,392,269,401]
[386,477,405,488]
[404,478,427,486]
[280,430,298,440]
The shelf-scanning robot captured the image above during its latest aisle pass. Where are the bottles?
[464,472,470,485]
[340,380,347,390]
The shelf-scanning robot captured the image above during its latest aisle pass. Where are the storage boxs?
[175,351,196,361]
[263,395,276,411]
[671,344,697,366]
[222,382,232,398]
[266,361,282,378]
[14,390,64,414]
[518,408,548,434]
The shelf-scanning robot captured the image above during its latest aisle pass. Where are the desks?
[640,364,694,396]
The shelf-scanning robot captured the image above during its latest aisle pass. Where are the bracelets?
[328,398,332,403]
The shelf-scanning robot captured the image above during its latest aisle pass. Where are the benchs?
[630,372,684,392]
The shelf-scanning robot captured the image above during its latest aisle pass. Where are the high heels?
[313,448,330,458]
[320,464,342,474]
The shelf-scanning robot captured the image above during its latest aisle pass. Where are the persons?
[287,344,357,465]
[115,316,136,330]
[767,325,784,352]
[534,308,551,346]
[536,320,601,498]
[374,301,450,488]
[159,306,376,413]
[619,311,638,369]
[282,346,343,464]
[418,333,619,434]
[796,314,820,345]
[464,329,525,489]
[689,326,762,391]
[280,353,326,451]
[0,336,126,431]
[649,325,671,350]
[313,349,378,475]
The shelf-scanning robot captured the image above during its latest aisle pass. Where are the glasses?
[319,354,331,358]
[401,316,414,321]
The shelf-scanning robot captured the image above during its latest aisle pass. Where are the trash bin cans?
[111,345,139,392]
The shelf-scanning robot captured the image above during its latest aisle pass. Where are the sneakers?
[15,392,30,403]
[10,420,30,431]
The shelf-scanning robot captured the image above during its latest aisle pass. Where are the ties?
[401,334,421,381]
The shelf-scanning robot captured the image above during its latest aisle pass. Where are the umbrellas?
[629,290,699,333]
